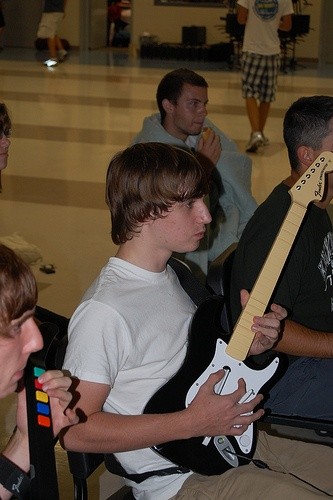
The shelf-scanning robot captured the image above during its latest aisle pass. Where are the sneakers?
[57,49,68,61]
[43,57,59,67]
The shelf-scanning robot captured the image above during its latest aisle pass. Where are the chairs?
[212,243,333,447]
[46,323,138,499]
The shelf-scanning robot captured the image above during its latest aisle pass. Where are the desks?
[222,13,311,72]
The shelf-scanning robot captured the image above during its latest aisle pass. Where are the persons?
[59,143,332,500]
[236,0,292,151]
[36,0,66,69]
[0,242,79,500]
[136,69,258,276]
[109,0,124,32]
[225,96,333,422]
[0,103,11,179]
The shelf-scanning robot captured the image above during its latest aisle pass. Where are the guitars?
[142,149,333,477]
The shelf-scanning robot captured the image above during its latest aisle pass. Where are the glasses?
[0,127,14,140]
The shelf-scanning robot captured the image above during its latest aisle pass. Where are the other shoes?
[264,136,269,144]
[245,131,265,151]
